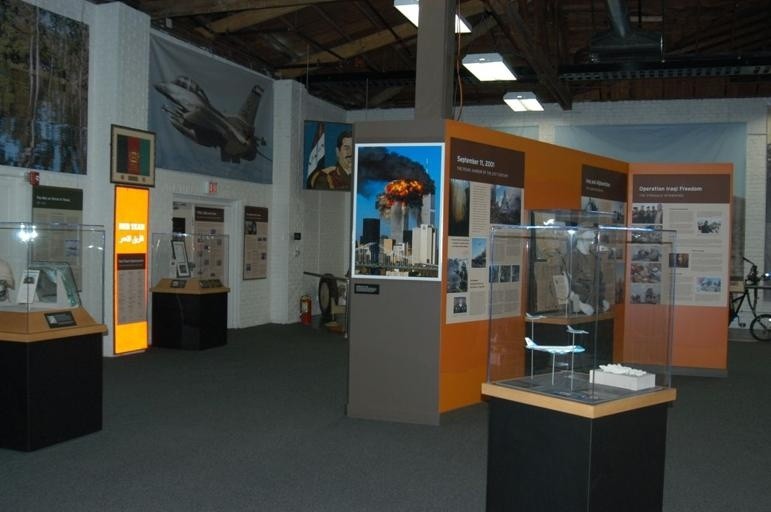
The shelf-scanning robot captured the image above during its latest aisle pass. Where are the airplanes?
[524,312,589,355]
[150,75,273,164]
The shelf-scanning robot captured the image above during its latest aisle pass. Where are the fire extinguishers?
[299,295,311,326]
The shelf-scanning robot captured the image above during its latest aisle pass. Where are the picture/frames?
[109,123,156,188]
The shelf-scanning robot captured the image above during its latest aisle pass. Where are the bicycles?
[728,257,771,342]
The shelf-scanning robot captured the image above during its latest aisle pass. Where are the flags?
[304,121,327,183]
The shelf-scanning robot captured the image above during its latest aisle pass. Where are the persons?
[306,130,354,189]
[632,201,665,227]
